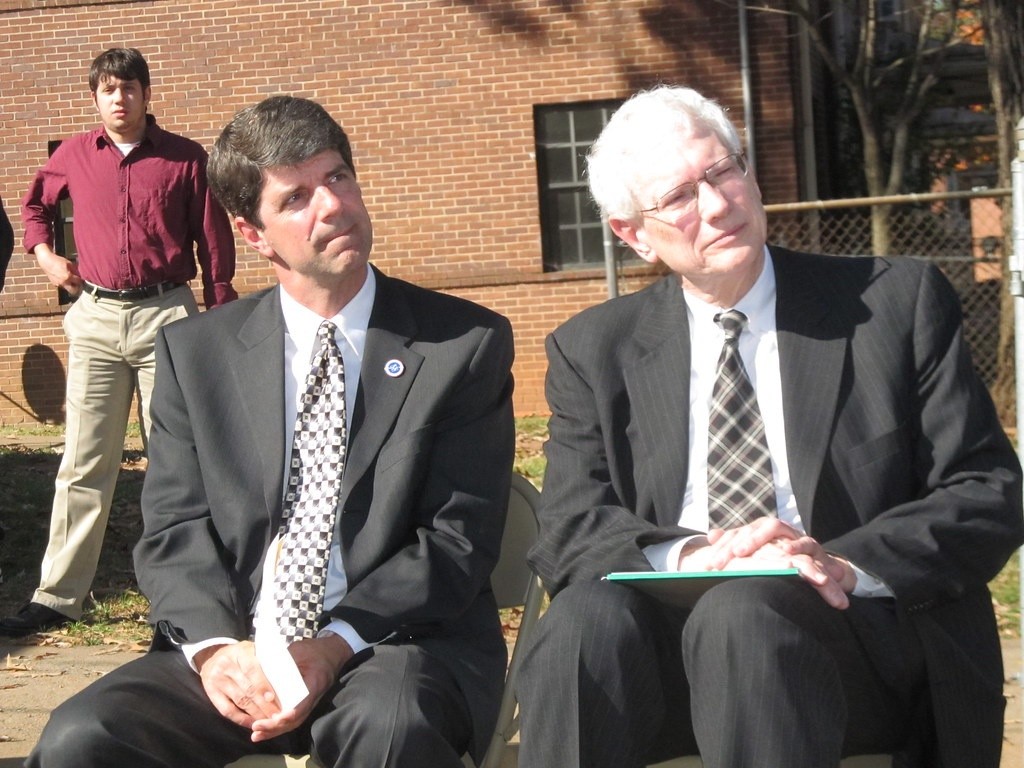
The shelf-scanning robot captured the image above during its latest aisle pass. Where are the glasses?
[640,152,748,220]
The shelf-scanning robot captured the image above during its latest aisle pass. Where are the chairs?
[224,474,542,767]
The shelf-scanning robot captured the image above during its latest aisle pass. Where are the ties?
[274,321,348,645]
[708,308,779,531]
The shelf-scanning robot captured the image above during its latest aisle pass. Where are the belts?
[81,279,186,302]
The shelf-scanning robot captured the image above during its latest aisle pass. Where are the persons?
[520,87,1020,766]
[1,42,243,634]
[21,98,519,765]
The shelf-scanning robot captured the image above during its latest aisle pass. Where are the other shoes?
[0,603,77,632]
